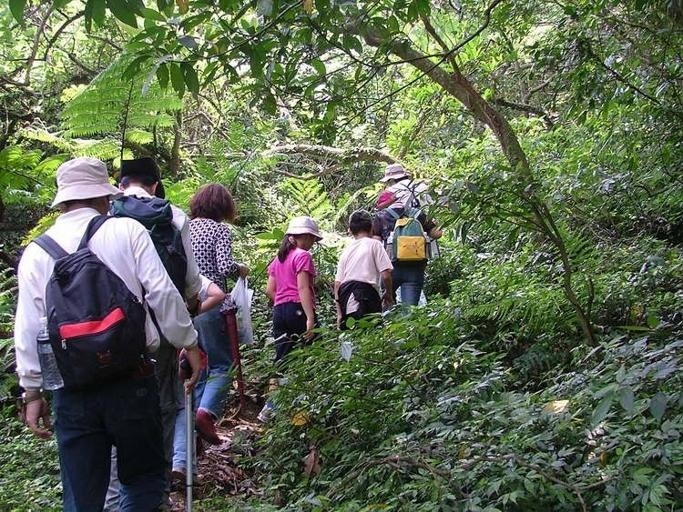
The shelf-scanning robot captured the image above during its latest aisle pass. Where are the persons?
[102,156,202,512]
[370,191,443,312]
[378,163,438,309]
[333,208,395,332]
[171,184,251,483]
[201,275,226,314]
[12,156,207,512]
[254,216,324,425]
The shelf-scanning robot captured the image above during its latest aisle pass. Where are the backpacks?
[112,195,187,297]
[34,227,146,391]
[384,206,427,262]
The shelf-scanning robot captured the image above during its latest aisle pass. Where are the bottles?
[36,323,64,391]
[423,232,432,259]
[386,232,396,262]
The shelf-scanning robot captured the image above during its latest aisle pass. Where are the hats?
[118,157,166,199]
[51,157,124,206]
[285,216,323,242]
[379,165,411,182]
[377,192,395,208]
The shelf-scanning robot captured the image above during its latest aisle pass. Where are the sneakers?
[257,404,275,424]
[194,407,221,448]
[170,472,198,499]
